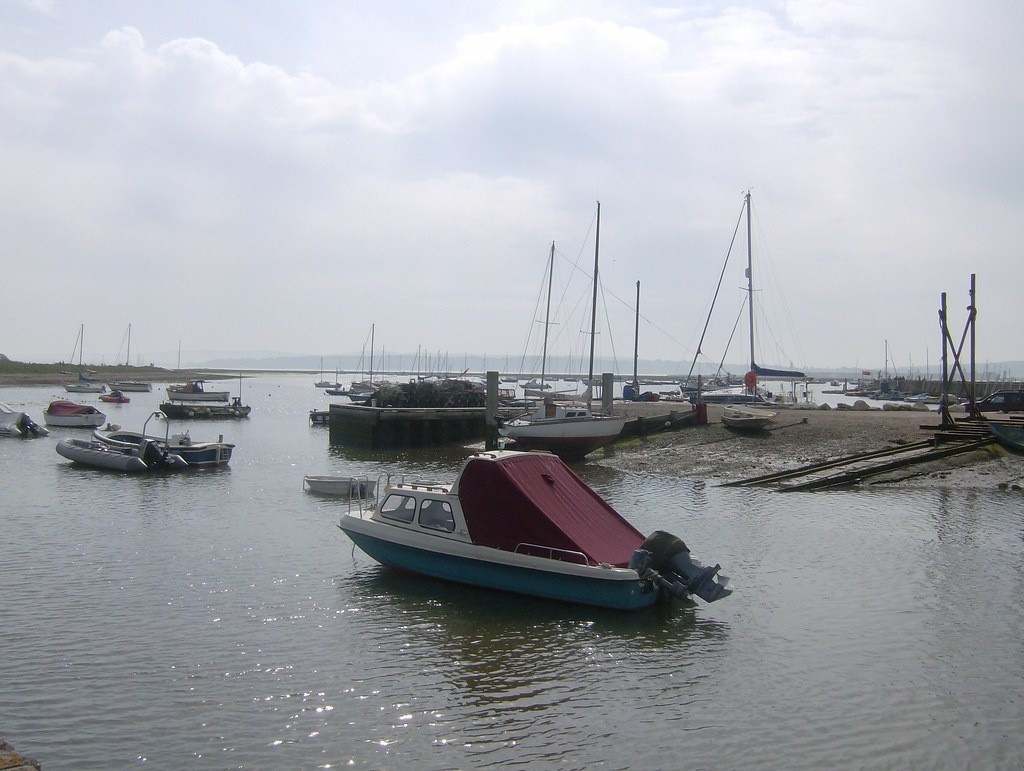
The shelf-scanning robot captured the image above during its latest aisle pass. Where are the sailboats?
[304,187,1024,459]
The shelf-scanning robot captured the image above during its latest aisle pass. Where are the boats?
[336,448,732,611]
[0,323,252,472]
[305,472,377,496]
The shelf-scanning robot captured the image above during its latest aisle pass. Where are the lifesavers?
[744,372,757,387]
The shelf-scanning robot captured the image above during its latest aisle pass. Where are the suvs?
[963,385,1024,412]
